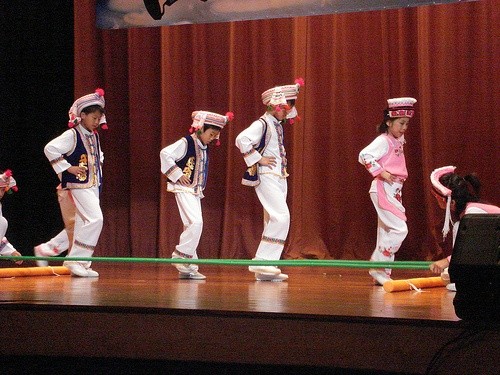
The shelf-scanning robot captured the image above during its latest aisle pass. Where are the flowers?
[295,76,304,87]
[96,87,105,98]
[3,170,13,180]
[226,110,235,122]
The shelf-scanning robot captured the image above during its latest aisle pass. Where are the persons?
[235,84,299,282]
[160,110,234,279]
[430,165,500,291]
[33,88,108,278]
[0,170,23,265]
[358,97,418,285]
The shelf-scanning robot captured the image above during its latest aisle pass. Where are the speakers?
[448,213,500,322]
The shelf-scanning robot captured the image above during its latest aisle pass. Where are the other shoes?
[249,266,288,280]
[369,270,392,284]
[170,262,206,279]
[35,247,49,267]
[446,282,457,292]
[63,262,99,277]
